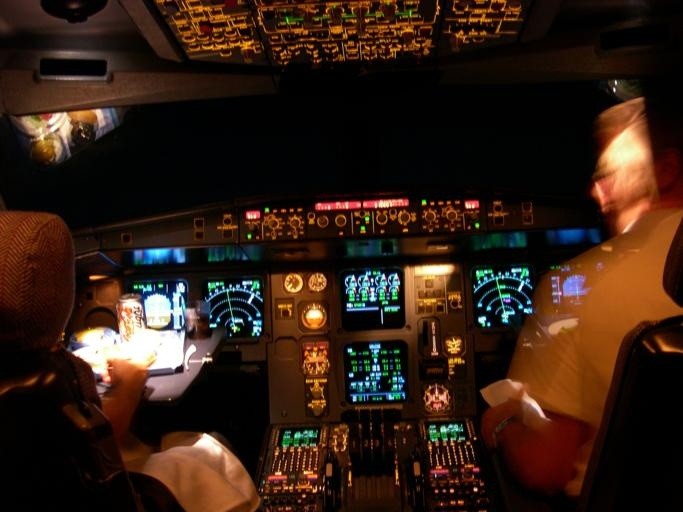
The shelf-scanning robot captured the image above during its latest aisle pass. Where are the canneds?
[115,292,148,342]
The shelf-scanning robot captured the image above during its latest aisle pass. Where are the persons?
[49,327,262,512]
[476,96,681,509]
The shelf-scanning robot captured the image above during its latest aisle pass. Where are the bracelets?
[489,417,522,452]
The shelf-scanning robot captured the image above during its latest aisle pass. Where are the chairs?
[577,211,681,511]
[1,211,188,510]
